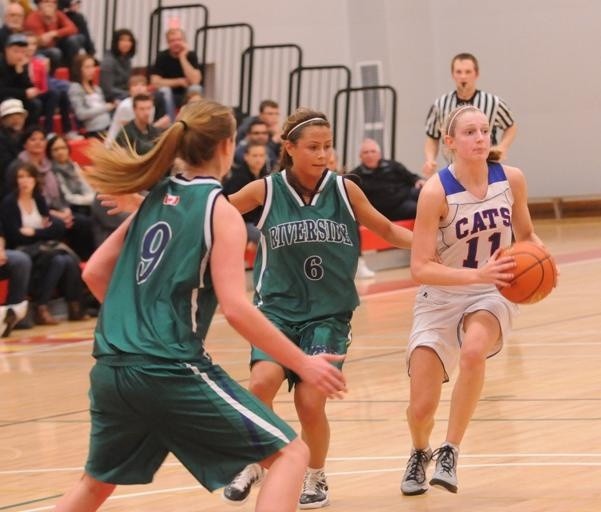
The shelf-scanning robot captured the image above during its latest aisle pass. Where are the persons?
[221,100,282,242]
[399,102,561,498]
[328,150,375,277]
[346,139,425,221]
[0,0,203,337]
[97,107,415,512]
[34,96,348,512]
[420,53,519,179]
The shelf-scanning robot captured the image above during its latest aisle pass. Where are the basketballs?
[494,239,556,304]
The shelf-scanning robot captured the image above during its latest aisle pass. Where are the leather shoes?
[17,301,91,329]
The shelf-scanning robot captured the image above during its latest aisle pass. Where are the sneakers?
[222,462,265,501]
[429,442,459,494]
[401,444,434,495]
[298,470,330,509]
[355,256,376,278]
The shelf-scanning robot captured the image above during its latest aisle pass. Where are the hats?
[0,98,28,119]
[6,33,29,46]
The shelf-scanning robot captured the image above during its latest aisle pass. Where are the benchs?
[244,219,414,269]
[0,259,87,309]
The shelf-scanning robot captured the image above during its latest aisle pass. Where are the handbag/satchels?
[23,239,66,268]
